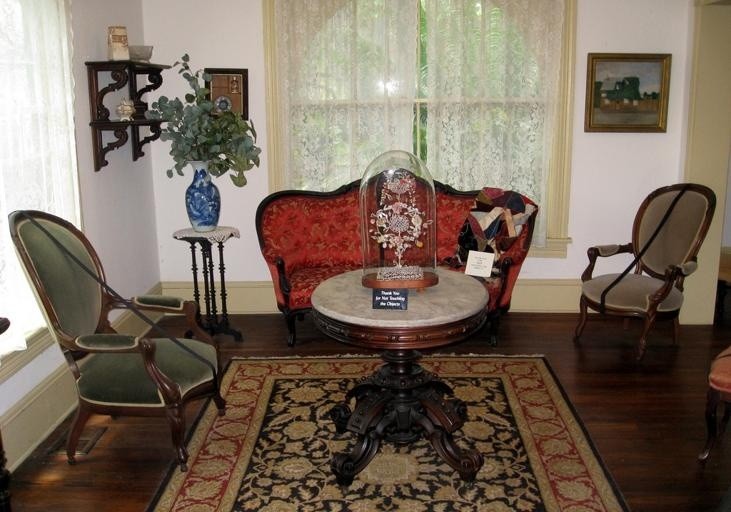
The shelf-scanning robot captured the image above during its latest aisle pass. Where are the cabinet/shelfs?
[84,60,172,172]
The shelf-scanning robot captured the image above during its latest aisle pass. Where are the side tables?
[174,226,241,343]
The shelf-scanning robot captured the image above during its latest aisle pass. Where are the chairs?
[699,344,731,460]
[7,209,226,471]
[573,183,716,362]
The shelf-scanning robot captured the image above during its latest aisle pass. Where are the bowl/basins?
[128,45,153,62]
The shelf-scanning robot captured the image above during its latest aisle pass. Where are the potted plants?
[145,54,260,231]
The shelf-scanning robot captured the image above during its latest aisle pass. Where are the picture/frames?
[205,68,248,121]
[584,52,672,132]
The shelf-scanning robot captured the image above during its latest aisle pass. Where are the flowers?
[369,169,432,279]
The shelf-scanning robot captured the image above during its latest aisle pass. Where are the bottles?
[107,26,131,60]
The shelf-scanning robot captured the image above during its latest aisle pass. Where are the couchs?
[254,177,540,347]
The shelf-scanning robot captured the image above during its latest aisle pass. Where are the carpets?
[144,354,630,512]
